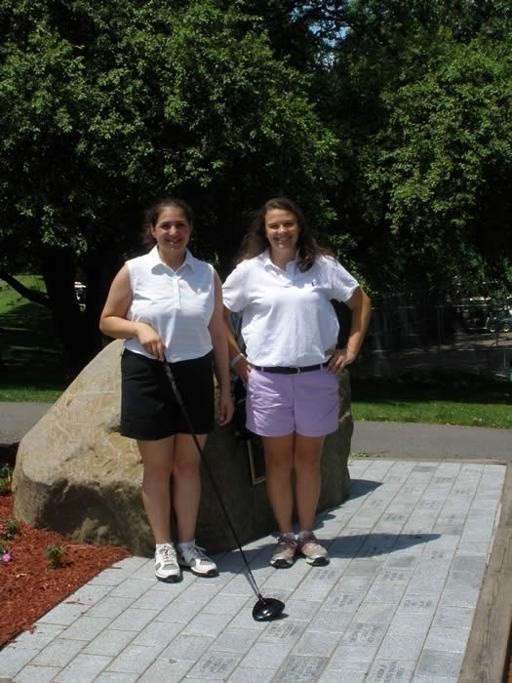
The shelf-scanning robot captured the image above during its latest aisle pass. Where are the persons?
[221,197,372,568]
[98,199,235,581]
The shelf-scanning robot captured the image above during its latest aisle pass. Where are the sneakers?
[154,544,218,582]
[269,534,329,568]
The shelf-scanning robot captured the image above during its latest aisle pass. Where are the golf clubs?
[158,354,286,622]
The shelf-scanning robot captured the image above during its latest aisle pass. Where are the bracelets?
[230,352,245,370]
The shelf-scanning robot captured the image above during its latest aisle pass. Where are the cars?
[466,306,512,333]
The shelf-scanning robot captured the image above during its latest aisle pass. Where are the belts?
[252,355,333,375]
